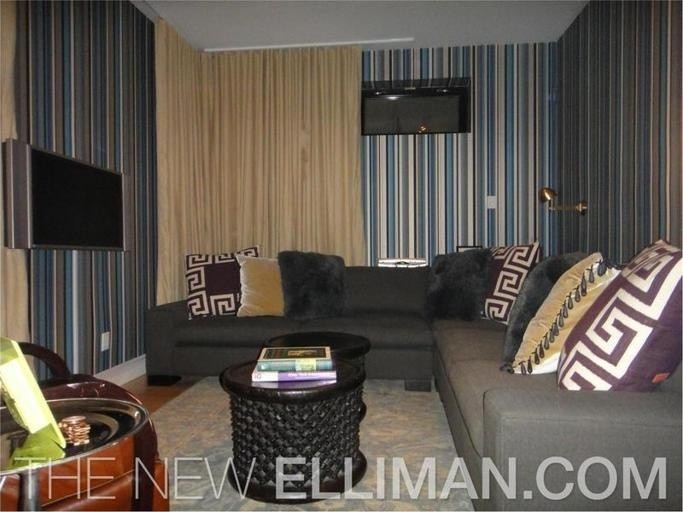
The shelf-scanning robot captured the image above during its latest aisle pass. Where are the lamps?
[538,188,588,215]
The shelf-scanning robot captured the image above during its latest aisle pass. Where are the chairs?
[0,342,169,512]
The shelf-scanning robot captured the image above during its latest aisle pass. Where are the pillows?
[186,247,259,322]
[236,255,285,318]
[278,251,352,320]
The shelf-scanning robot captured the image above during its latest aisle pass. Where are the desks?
[0,397,149,512]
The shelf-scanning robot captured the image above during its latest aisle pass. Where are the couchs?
[144,266,436,391]
[431,318,683,512]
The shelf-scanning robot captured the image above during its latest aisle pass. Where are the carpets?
[149,375,475,512]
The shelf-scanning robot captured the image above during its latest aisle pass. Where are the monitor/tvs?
[3,139,126,250]
